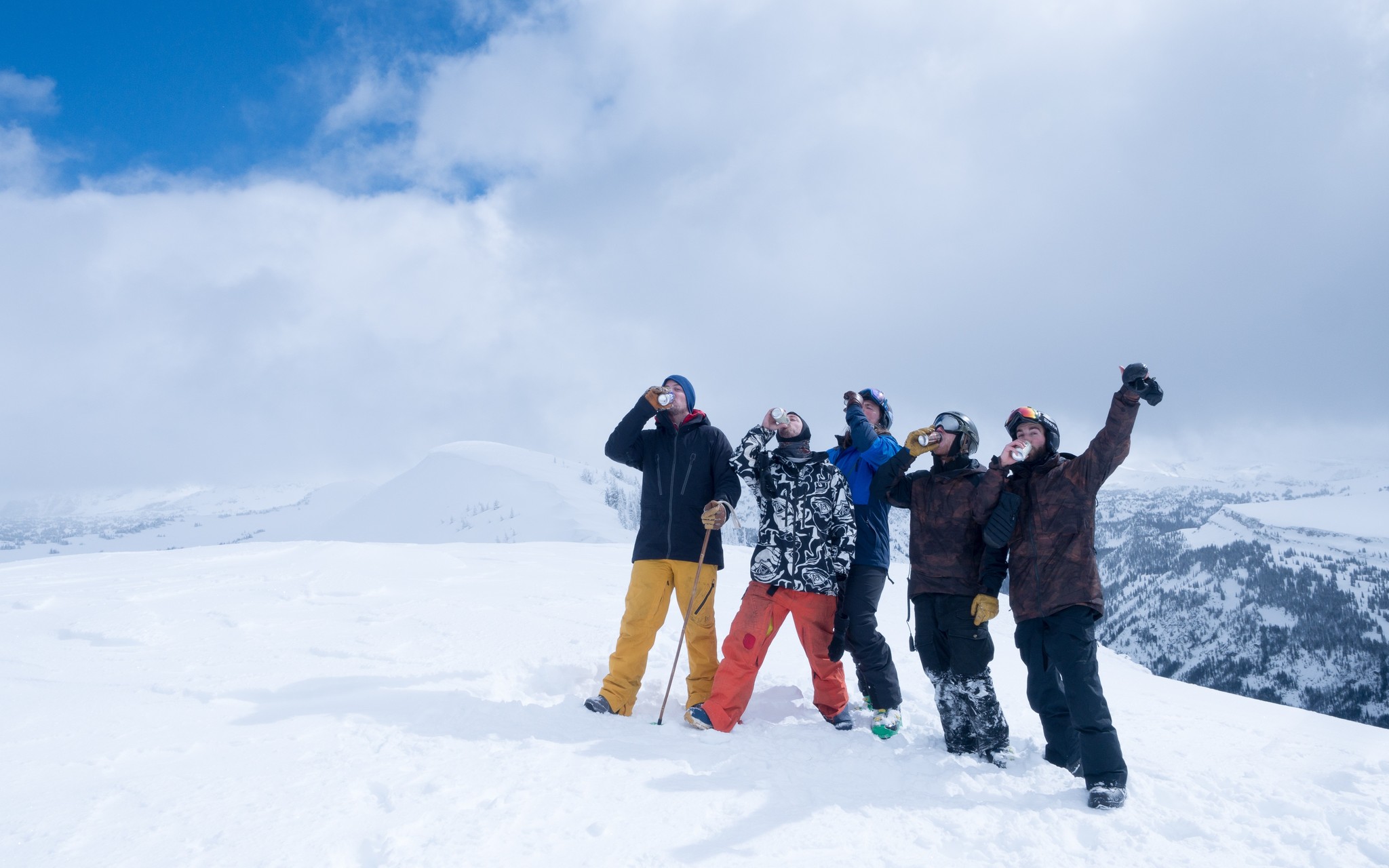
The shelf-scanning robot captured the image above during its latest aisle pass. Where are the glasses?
[933,413,964,435]
[858,388,884,405]
[1004,407,1039,428]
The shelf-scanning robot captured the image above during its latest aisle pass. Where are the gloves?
[827,612,849,662]
[701,500,726,530]
[984,491,1022,549]
[844,391,864,413]
[1122,363,1163,406]
[904,424,940,457]
[758,451,778,500]
[642,386,674,411]
[971,594,999,626]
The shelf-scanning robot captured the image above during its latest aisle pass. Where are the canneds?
[918,432,943,446]
[657,391,675,406]
[1011,440,1032,461]
[771,408,791,425]
[844,399,849,407]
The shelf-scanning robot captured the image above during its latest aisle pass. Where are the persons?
[870,411,1012,770]
[825,387,905,740]
[583,374,742,717]
[684,407,858,733]
[972,364,1150,811]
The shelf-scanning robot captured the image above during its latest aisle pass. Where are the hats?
[661,375,696,414]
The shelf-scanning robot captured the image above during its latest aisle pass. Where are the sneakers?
[1089,786,1126,811]
[694,703,704,708]
[684,707,714,730]
[584,695,620,714]
[831,703,853,730]
[854,695,873,711]
[986,744,1016,768]
[871,704,902,738]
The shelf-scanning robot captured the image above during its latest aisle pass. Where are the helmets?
[858,391,893,430]
[1008,411,1059,454]
[930,411,979,458]
